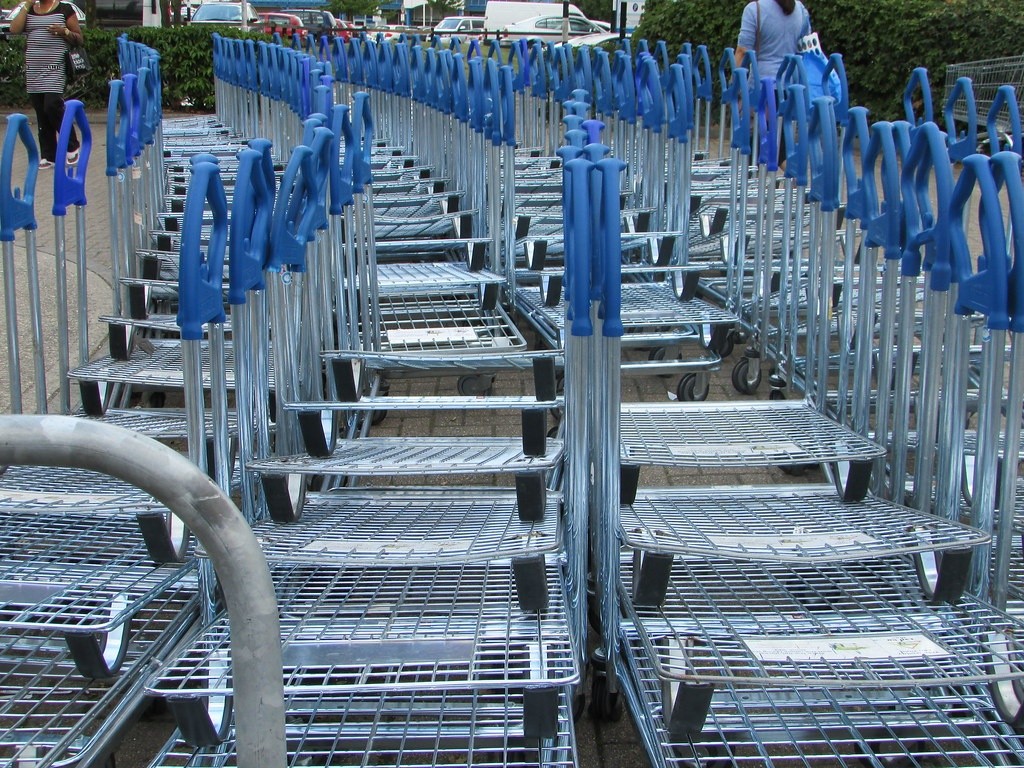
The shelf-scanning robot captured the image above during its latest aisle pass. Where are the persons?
[9,0,84,169]
[729,0,813,191]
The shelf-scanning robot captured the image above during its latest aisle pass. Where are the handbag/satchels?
[798,3,843,107]
[736,97,769,137]
[67,34,93,76]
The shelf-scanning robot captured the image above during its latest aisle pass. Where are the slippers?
[67,149,80,164]
[37,159,55,170]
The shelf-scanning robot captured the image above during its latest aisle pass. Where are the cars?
[0,0,86,34]
[256,12,306,38]
[335,17,352,43]
[370,24,409,39]
[343,20,360,38]
[170,5,196,22]
[189,1,262,29]
[543,31,634,51]
[62,0,142,27]
[500,14,610,50]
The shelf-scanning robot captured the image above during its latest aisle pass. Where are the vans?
[426,15,484,42]
[483,0,592,44]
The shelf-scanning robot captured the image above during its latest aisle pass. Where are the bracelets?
[63,27,69,37]
[24,4,30,12]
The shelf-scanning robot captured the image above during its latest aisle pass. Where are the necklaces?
[38,0,55,13]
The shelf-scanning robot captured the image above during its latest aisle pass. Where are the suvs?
[281,9,338,44]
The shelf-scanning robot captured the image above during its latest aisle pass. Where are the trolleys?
[939,52,1024,156]
[0,28,1024,768]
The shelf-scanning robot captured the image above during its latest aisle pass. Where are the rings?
[55,31,57,34]
